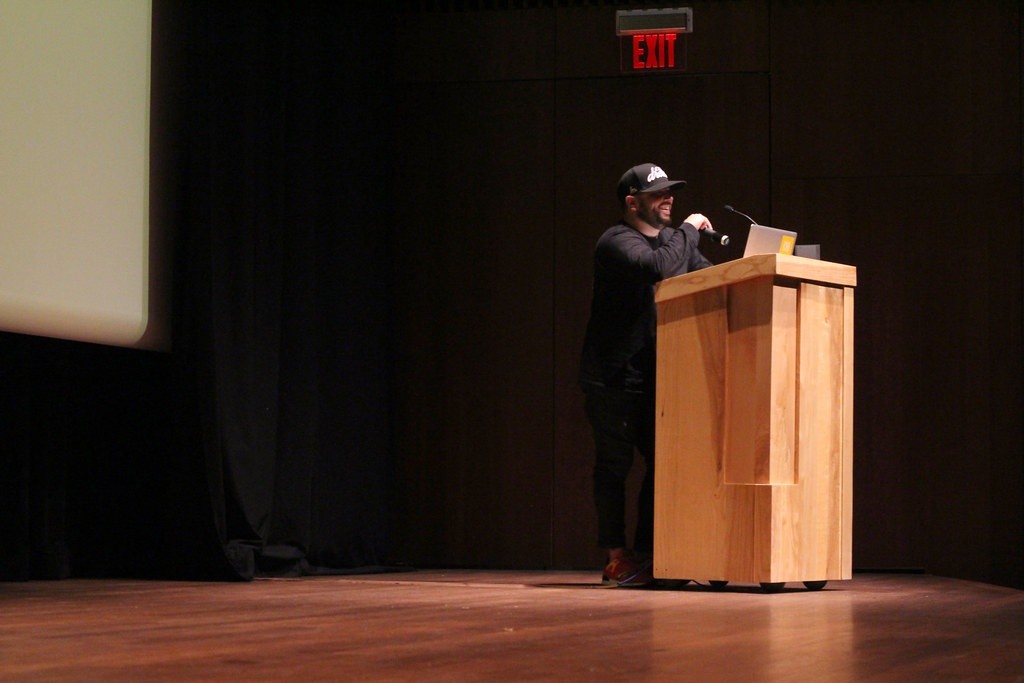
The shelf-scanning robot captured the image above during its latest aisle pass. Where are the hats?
[615,163,687,202]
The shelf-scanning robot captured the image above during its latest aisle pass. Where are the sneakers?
[601,554,656,589]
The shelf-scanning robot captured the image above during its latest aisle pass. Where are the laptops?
[742,224,797,259]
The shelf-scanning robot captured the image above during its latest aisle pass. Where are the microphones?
[724,205,758,225]
[699,227,730,247]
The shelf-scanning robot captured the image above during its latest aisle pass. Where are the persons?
[579,163,712,588]
